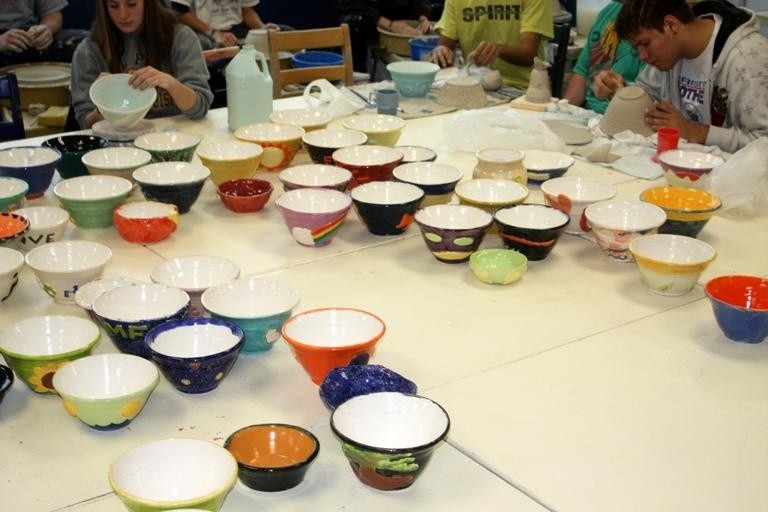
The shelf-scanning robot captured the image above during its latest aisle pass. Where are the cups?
[658,127,679,159]
[377,88,399,114]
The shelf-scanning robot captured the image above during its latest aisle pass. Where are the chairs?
[0,74,26,143]
[546,22,570,98]
[204,46,240,109]
[265,24,351,99]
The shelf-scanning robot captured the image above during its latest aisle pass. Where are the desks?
[0,66,768,511]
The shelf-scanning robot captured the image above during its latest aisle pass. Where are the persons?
[0,0,92,67]
[428,0,555,93]
[594,0,768,151]
[69,0,214,129]
[358,0,431,84]
[167,0,294,52]
[560,0,648,120]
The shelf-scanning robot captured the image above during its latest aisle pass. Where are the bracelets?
[210,28,216,37]
[389,21,392,32]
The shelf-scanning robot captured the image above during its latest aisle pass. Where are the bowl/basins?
[330,391,450,490]
[659,149,724,181]
[0,247,25,302]
[132,160,210,213]
[11,206,69,250]
[468,250,528,284]
[0,213,30,250]
[413,205,493,264]
[223,423,320,491]
[54,175,132,229]
[292,52,343,85]
[332,145,404,190]
[523,150,575,183]
[281,308,386,385]
[42,135,105,178]
[90,73,157,131]
[53,353,159,429]
[629,234,715,296]
[91,120,156,141]
[270,110,333,151]
[144,317,244,393]
[75,278,143,327]
[409,36,438,60]
[639,187,722,237]
[114,201,179,242]
[342,114,406,147]
[599,87,657,138]
[134,131,199,162]
[392,162,461,194]
[244,29,274,56]
[350,182,424,235]
[196,139,263,186]
[386,61,440,97]
[0,146,62,200]
[438,78,488,109]
[454,179,529,233]
[26,241,113,304]
[302,130,368,164]
[585,200,667,263]
[0,363,14,403]
[705,276,768,344]
[395,145,437,162]
[108,435,239,512]
[492,204,570,260]
[235,123,305,172]
[0,316,101,394]
[201,278,300,354]
[275,187,351,247]
[216,178,272,213]
[279,163,353,191]
[150,255,240,316]
[0,175,29,211]
[542,179,615,235]
[473,149,528,185]
[82,146,151,191]
[1,61,72,112]
